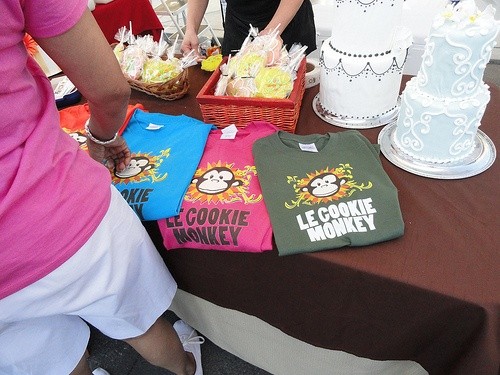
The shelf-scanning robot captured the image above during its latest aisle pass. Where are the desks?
[45,53,500,375]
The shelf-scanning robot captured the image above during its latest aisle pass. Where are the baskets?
[110,43,191,101]
[195,56,306,134]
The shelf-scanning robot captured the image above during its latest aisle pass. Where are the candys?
[113,21,200,83]
[213,23,308,99]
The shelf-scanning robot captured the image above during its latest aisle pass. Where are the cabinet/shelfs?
[154,0,221,53]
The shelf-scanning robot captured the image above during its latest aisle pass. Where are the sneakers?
[173,320,205,375]
[92,367,110,375]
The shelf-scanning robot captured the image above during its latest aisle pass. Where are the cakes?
[393,0,500,165]
[318,0,411,119]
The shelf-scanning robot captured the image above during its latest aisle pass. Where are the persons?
[0,0,203,375]
[180,0,317,62]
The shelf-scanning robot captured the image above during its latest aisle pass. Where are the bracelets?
[84,118,118,144]
